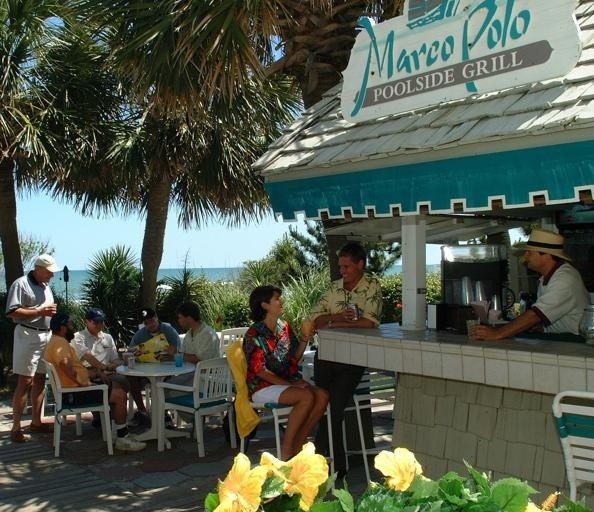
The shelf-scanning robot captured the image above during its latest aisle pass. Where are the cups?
[121,352,133,367]
[174,352,183,368]
[48,303,57,312]
[466,318,481,339]
[461,275,504,321]
[159,352,169,366]
[302,319,316,337]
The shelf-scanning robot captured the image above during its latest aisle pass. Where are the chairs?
[552,391,594,502]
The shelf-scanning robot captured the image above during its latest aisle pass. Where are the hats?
[512,228,572,262]
[138,306,156,322]
[34,254,61,274]
[85,308,107,322]
[51,311,77,332]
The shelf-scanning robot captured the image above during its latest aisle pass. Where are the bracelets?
[327,320,336,329]
[299,335,309,344]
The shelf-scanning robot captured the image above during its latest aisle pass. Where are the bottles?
[578,303,594,347]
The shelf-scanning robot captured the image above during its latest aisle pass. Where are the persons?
[242,285,331,462]
[154,301,221,426]
[44,313,147,452]
[308,243,383,481]
[69,307,131,431]
[5,254,60,443]
[127,307,181,427]
[468,227,590,343]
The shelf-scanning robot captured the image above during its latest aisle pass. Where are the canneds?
[128,358,134,369]
[347,303,359,321]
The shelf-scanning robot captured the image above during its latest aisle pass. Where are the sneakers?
[91,418,102,428]
[29,422,53,434]
[9,429,28,443]
[114,435,147,452]
[163,410,174,428]
[127,410,149,432]
